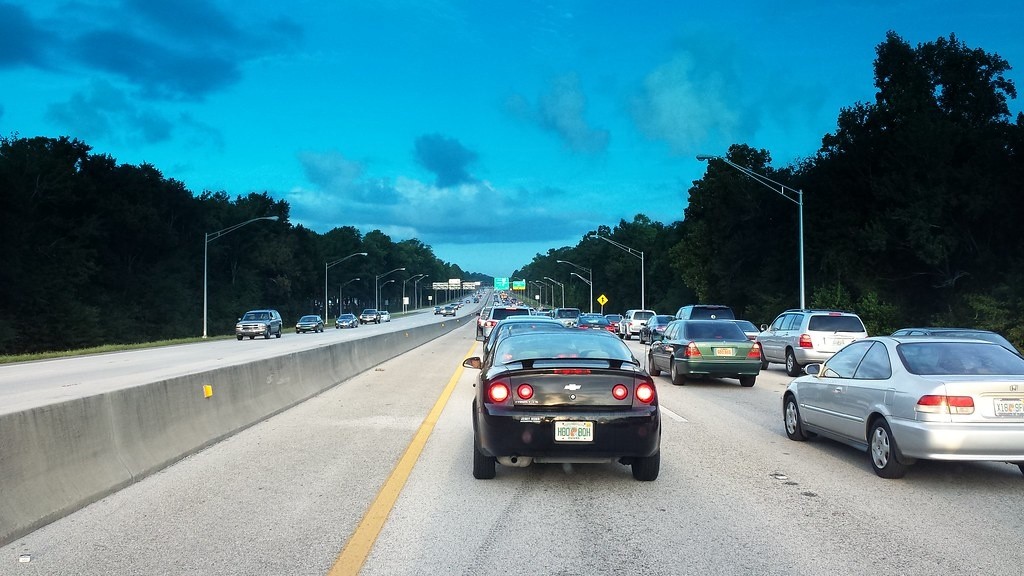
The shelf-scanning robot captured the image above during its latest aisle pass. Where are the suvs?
[236,309,282,341]
[754,309,869,377]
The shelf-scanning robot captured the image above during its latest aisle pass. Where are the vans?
[672,305,736,320]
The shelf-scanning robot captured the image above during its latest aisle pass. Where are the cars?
[618,310,657,340]
[781,335,1024,480]
[461,327,662,482]
[573,316,614,333]
[450,291,487,310]
[378,311,391,322]
[605,315,624,332]
[639,315,676,346]
[718,319,760,344]
[433,307,444,315]
[442,307,456,317]
[476,291,603,343]
[335,314,358,329]
[646,320,763,388]
[295,315,325,334]
[889,327,1023,359]
[359,309,381,325]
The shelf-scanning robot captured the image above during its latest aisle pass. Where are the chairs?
[580,349,610,363]
[249,316,255,320]
[598,320,604,323]
[518,350,540,360]
[581,319,588,323]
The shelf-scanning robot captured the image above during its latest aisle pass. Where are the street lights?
[340,278,361,315]
[379,280,395,311]
[557,260,592,281]
[376,267,406,310]
[403,274,423,311]
[536,280,554,307]
[544,276,565,308]
[590,234,645,310]
[325,253,368,324]
[570,272,593,313]
[696,154,805,311]
[415,275,429,310]
[202,215,279,339]
[529,281,541,307]
